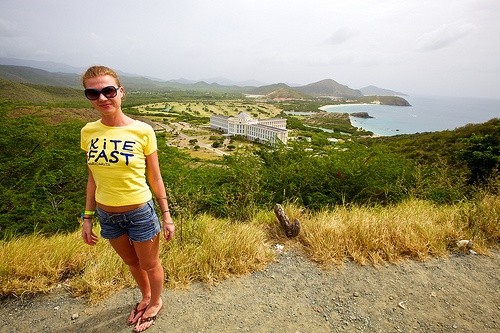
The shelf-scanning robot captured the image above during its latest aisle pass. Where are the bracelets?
[161,209,170,213]
[83,217,93,219]
[163,219,174,225]
[84,210,95,215]
[156,196,168,199]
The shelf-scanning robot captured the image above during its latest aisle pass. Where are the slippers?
[133,301,163,333]
[126,300,151,326]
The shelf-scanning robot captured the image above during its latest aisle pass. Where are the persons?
[82,66,175,333]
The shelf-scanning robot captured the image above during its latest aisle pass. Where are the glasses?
[84,86,121,100]
